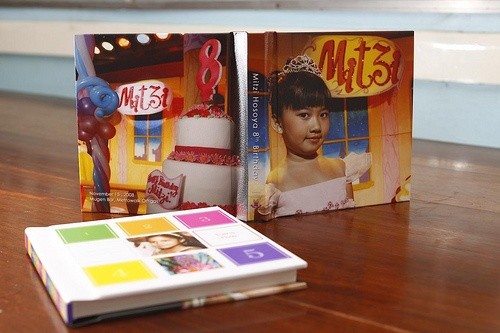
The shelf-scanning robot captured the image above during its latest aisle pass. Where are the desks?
[0,90,500,333]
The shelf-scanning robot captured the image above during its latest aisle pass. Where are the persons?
[127,233,208,257]
[250,55,357,222]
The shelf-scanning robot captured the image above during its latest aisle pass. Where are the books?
[74,28,415,222]
[23,206,308,327]
